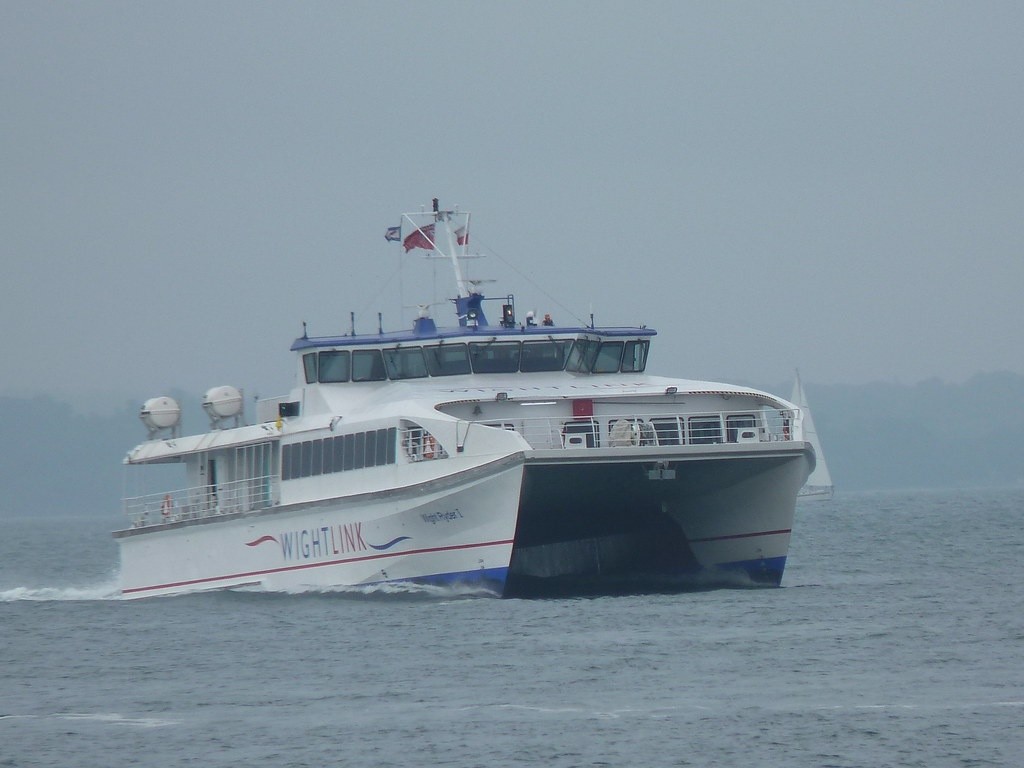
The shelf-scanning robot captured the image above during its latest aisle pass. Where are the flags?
[385,226,401,241]
[455,222,469,245]
[404,223,435,254]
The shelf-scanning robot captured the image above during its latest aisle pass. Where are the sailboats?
[796,374,834,498]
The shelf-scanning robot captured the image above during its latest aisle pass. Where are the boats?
[98,195,816,604]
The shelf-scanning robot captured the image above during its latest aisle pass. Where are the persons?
[526,309,538,326]
[424,435,434,458]
[783,416,793,441]
[542,314,554,325]
